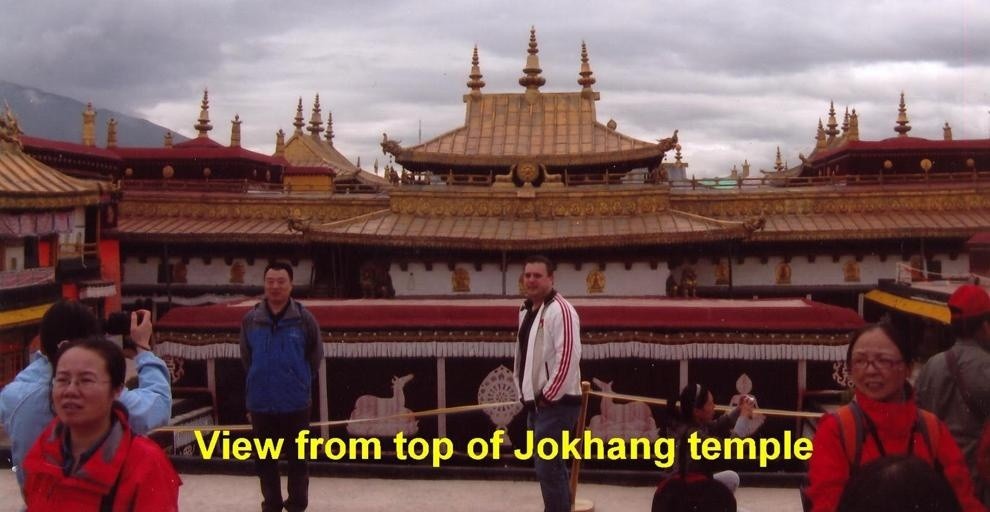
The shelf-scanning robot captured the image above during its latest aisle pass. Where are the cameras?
[745,394,754,401]
[107,311,142,335]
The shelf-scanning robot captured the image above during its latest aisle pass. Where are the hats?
[948,283,989,320]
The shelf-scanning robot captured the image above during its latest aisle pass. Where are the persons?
[514,258,588,512]
[1,302,172,512]
[239,261,323,512]
[910,280,989,511]
[668,383,756,492]
[804,319,989,512]
[13,335,184,511]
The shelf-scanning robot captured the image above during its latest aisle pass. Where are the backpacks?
[835,402,963,512]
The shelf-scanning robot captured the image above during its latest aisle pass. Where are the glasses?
[849,356,907,369]
